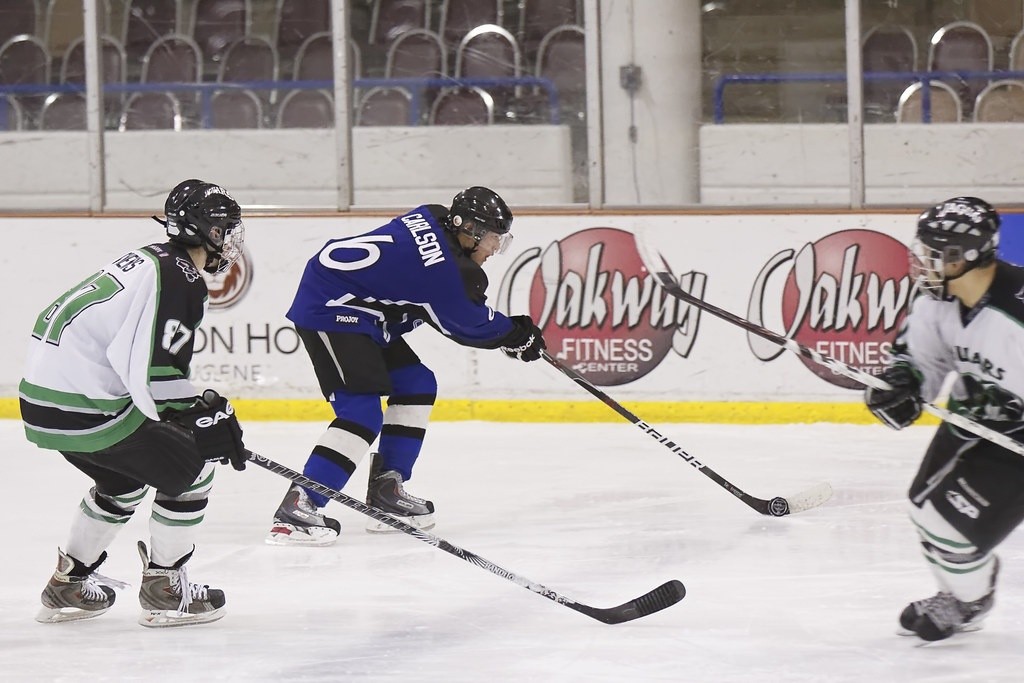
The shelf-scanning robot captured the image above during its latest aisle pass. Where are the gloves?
[948,385,1024,440]
[865,366,922,431]
[184,389,246,471]
[500,315,547,362]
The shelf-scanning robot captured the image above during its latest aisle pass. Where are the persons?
[264,186,548,546]
[863,197,1024,648]
[20,179,249,629]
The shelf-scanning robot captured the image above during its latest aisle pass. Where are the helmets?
[151,179,245,276]
[907,196,1002,300]
[443,186,514,257]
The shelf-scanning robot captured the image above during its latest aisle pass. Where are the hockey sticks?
[634,232,1024,456]
[539,349,832,518]
[242,447,686,626]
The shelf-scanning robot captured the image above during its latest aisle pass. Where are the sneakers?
[264,481,341,547]
[137,540,226,628]
[901,555,1001,643]
[365,453,435,532]
[34,546,116,623]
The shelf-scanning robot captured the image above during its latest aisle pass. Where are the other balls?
[768,497,787,516]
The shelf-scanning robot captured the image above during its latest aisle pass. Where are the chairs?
[0,0,1024,136]
